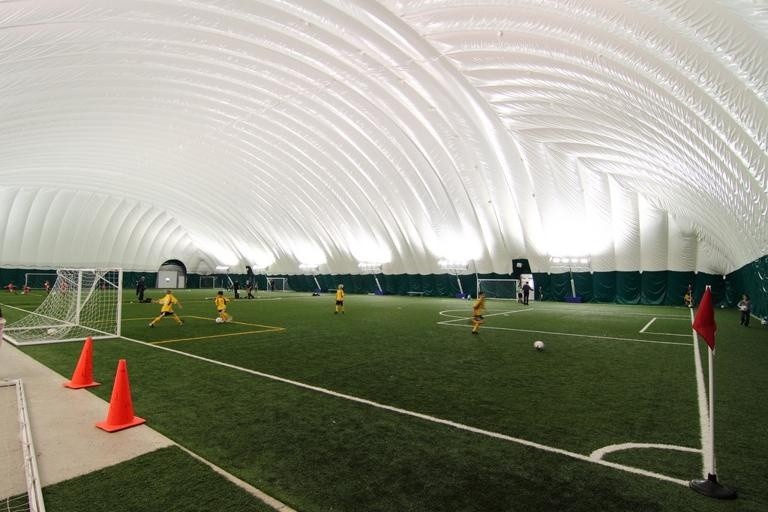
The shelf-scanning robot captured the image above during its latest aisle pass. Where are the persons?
[521,281,533,306]
[203,290,231,323]
[21,285,30,295]
[43,279,50,295]
[146,288,185,329]
[736,293,752,327]
[270,279,275,292]
[229,278,241,299]
[516,283,522,303]
[333,284,345,315]
[470,291,488,336]
[245,279,255,300]
[3,282,17,293]
[135,275,147,303]
[59,279,69,298]
[683,292,695,307]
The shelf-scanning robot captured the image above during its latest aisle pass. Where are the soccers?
[166,278,169,282]
[216,317,222,323]
[48,329,56,335]
[534,340,544,350]
[762,320,766,325]
[721,305,724,308]
[741,306,747,311]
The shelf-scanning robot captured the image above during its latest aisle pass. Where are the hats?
[338,284,344,289]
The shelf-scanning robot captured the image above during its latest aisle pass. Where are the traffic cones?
[95,359,149,434]
[62,334,106,389]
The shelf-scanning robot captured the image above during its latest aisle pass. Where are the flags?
[690,287,719,355]
